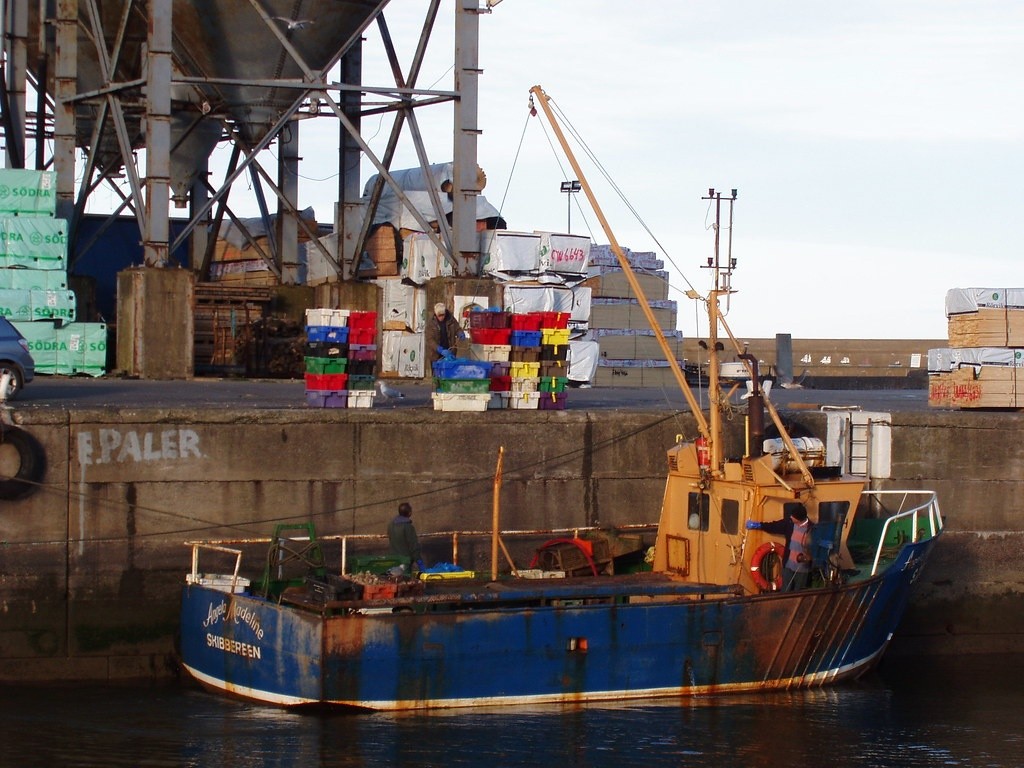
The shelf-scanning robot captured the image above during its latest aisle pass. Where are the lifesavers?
[0,424,47,503]
[750,541,786,589]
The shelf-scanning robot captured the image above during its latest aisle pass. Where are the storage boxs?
[511,568,566,580]
[302,570,475,602]
[431,311,572,412]
[305,309,377,408]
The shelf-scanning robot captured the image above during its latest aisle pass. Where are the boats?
[179,186,945,711]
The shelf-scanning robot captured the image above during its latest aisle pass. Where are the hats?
[434,303,445,316]
[790,503,807,521]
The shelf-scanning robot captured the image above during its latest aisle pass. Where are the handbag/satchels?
[746,521,761,529]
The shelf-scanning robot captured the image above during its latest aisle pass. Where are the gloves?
[459,332,465,340]
[438,347,451,356]
[416,559,423,565]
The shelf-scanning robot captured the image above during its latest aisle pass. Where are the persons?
[387,502,425,574]
[746,503,816,592]
[427,303,465,385]
